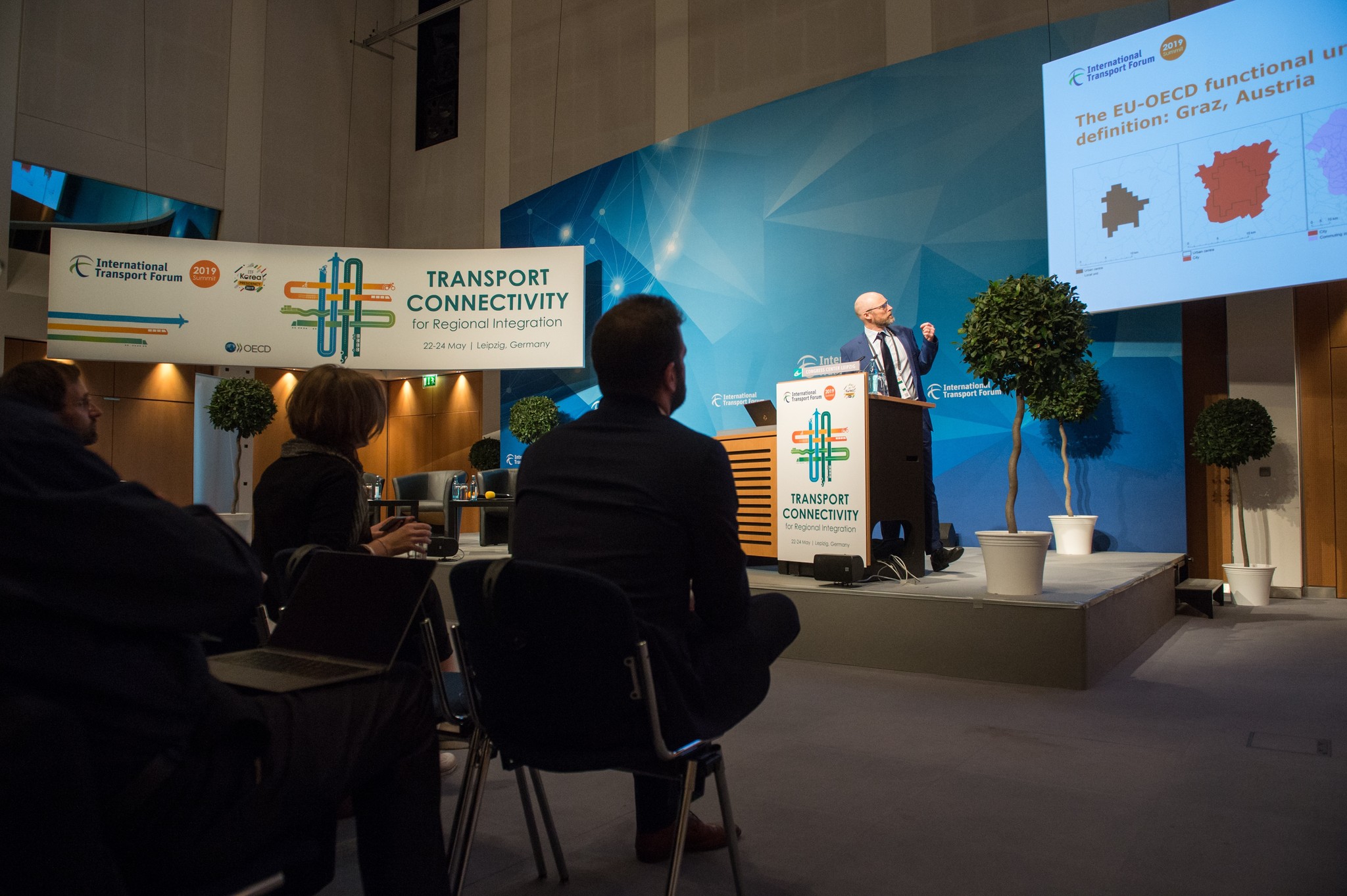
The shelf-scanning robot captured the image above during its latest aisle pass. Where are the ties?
[876,332,903,399]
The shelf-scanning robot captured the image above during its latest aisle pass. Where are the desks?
[448,498,516,554]
[369,498,419,523]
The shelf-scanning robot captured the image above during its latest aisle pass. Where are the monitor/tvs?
[743,400,777,427]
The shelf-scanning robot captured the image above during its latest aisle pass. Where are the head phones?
[285,543,331,595]
[480,553,514,632]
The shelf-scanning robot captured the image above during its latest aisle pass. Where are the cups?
[459,484,468,500]
[365,483,372,500]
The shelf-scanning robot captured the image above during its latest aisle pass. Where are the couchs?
[476,470,519,546]
[392,471,466,541]
[362,472,386,527]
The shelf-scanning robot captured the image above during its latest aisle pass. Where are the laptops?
[199,549,438,694]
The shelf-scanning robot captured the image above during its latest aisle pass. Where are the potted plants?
[1024,358,1104,556]
[1187,396,1278,608]
[949,273,1098,596]
[202,377,278,544]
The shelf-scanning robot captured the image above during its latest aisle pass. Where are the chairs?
[0,505,771,896]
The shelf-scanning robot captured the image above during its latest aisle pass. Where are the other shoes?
[439,752,457,776]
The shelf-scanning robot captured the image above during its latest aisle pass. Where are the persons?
[251,362,458,782]
[840,291,964,572]
[1,400,458,896]
[510,292,801,863]
[0,361,102,448]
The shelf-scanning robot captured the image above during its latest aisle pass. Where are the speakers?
[813,553,864,586]
[426,536,459,562]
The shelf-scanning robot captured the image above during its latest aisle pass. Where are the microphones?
[836,356,865,376]
[863,354,877,372]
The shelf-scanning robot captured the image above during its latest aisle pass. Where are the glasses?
[865,300,889,312]
[65,394,94,410]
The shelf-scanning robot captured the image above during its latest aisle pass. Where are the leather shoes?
[635,810,742,864]
[931,546,964,572]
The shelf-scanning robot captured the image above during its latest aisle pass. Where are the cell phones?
[379,515,410,531]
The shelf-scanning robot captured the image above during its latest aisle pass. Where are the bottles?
[878,372,884,395]
[470,475,478,501]
[869,359,878,393]
[374,475,382,501]
[452,475,459,500]
